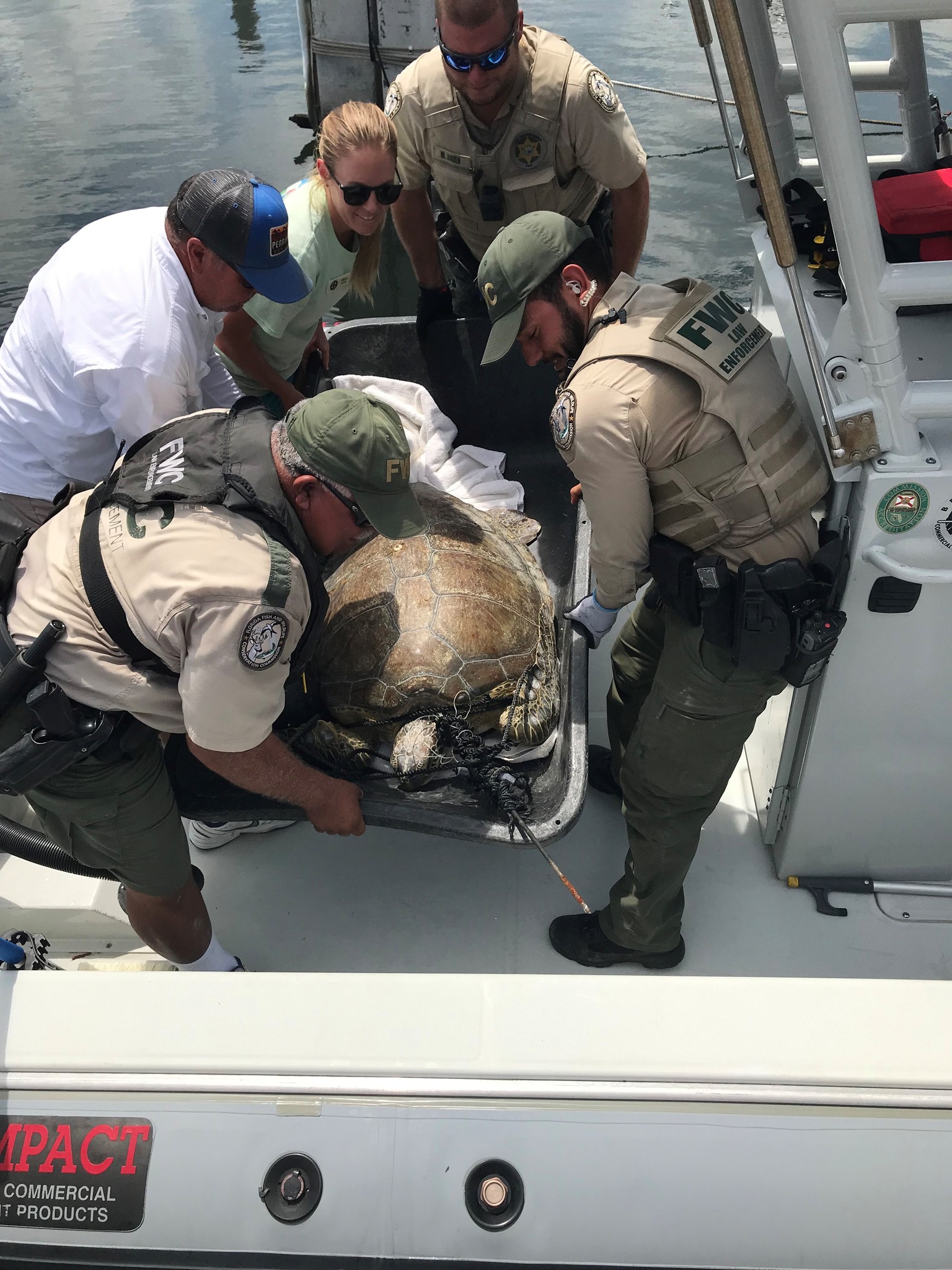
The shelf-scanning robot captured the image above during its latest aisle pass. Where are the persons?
[379,0,650,519]
[0,164,312,620]
[213,95,404,426]
[0,386,430,978]
[475,205,835,971]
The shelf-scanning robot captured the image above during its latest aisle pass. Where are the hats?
[178,167,314,304]
[477,211,595,366]
[284,387,430,542]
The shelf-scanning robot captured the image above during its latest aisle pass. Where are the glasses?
[321,150,404,206]
[438,14,516,73]
[281,460,372,529]
[218,255,255,289]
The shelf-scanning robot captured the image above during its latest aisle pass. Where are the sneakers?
[547,911,687,970]
[585,744,624,798]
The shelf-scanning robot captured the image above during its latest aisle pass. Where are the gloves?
[416,282,455,324]
[560,588,625,650]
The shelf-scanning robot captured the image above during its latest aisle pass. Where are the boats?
[0,0,952,1270]
